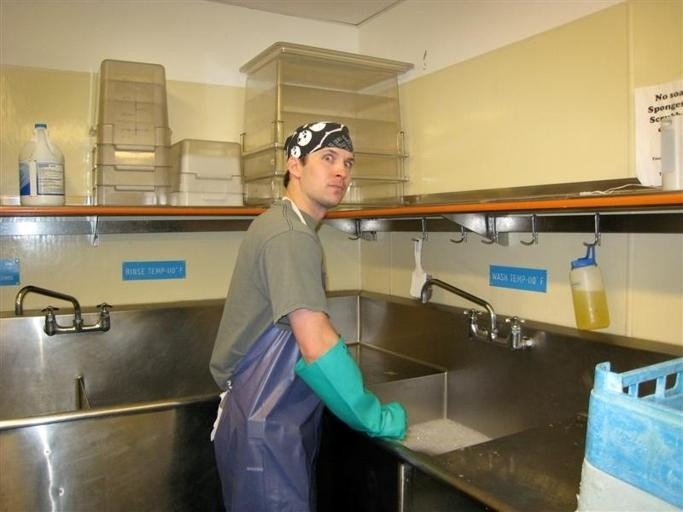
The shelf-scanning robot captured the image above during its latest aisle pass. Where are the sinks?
[361,371,583,459]
[0,296,448,424]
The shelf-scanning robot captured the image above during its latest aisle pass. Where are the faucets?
[15,285,112,336]
[420,278,537,354]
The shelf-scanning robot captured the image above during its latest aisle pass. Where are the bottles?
[18,123,66,208]
[568,243,610,332]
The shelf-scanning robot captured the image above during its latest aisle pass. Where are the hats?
[289,122,354,157]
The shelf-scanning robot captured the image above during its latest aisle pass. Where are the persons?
[210,120,408,512]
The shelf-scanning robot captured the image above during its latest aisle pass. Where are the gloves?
[293,336,407,442]
